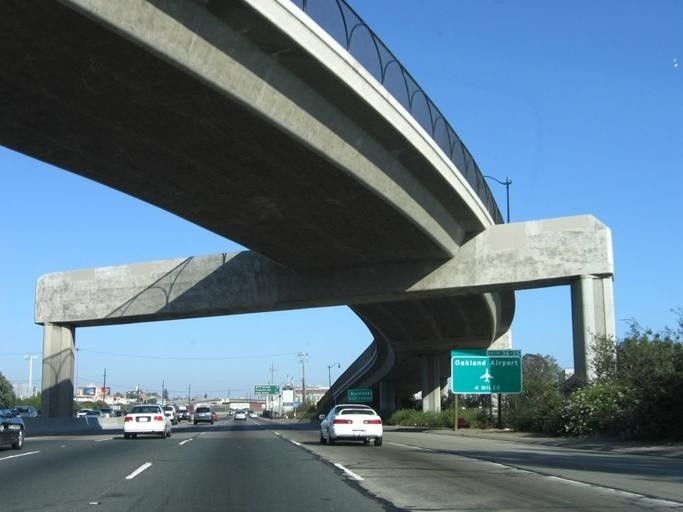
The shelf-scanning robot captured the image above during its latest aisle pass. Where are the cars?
[191,404,214,425]
[123,403,172,438]
[233,411,245,420]
[0,402,38,450]
[76,405,190,422]
[317,403,384,444]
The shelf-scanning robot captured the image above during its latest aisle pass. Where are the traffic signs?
[448,348,522,395]
